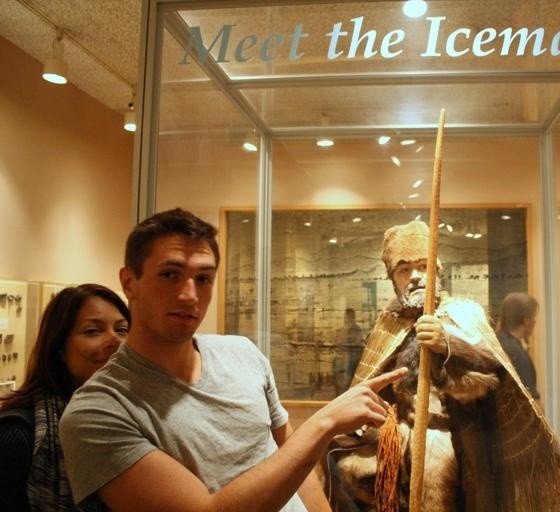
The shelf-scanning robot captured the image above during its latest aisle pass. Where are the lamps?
[123,87,136,132]
[42,28,69,85]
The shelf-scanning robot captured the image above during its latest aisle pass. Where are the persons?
[0,283,132,511]
[333,306,361,397]
[58,205,409,511]
[309,218,560,512]
[495,292,539,400]
